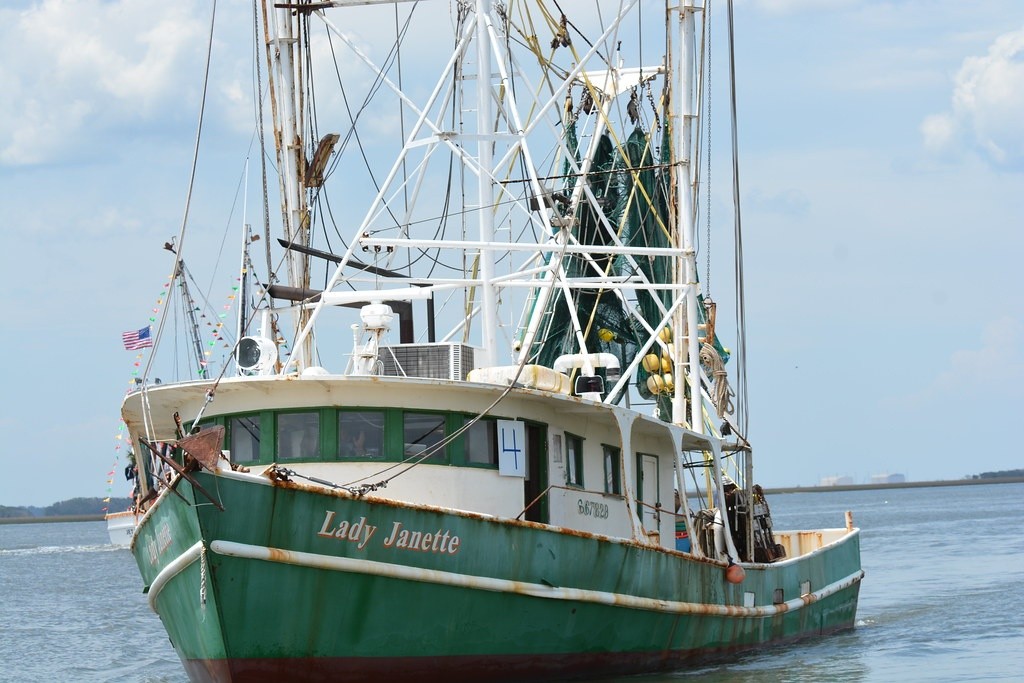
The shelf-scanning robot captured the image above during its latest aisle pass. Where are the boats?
[106,0,865,683]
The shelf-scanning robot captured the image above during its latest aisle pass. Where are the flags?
[122,325,153,349]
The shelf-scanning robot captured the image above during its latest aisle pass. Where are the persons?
[719,475,746,554]
